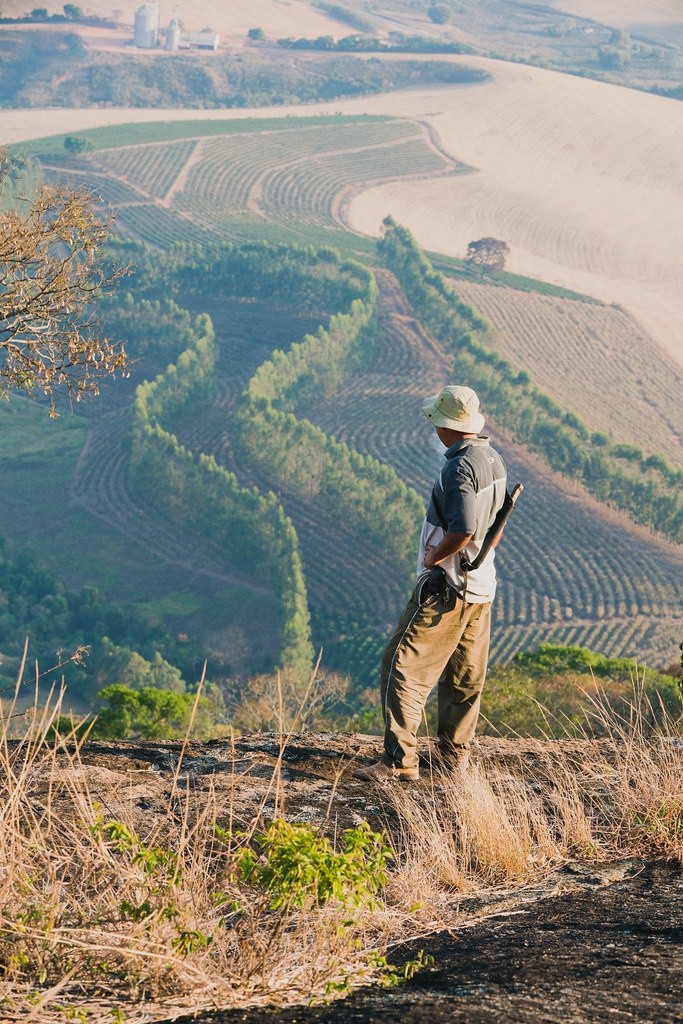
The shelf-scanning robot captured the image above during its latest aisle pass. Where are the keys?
[443,587,451,608]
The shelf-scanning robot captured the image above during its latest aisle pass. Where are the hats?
[422,386,486,433]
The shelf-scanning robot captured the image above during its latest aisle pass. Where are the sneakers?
[418,742,471,771]
[352,760,420,780]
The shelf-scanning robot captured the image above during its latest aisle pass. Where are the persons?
[352,386,508,782]
